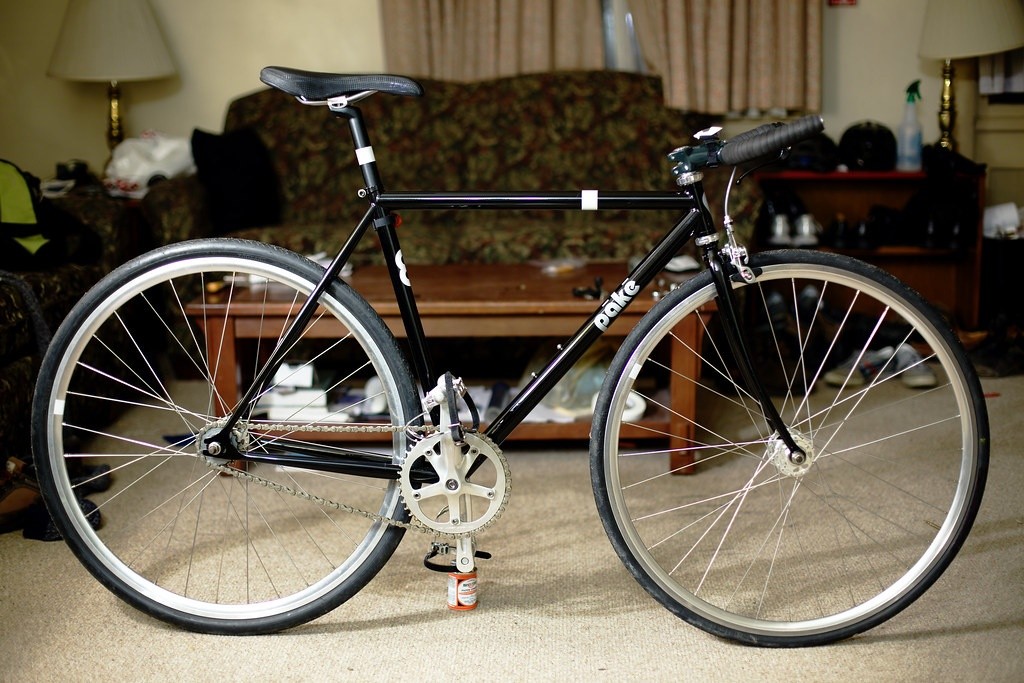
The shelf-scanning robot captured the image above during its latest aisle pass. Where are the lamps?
[916,0,1024,151]
[46,0,178,152]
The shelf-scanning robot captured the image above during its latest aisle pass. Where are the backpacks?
[0,159,104,273]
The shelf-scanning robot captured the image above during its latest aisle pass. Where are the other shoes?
[968,314,1024,377]
[853,222,875,250]
[792,213,823,246]
[819,220,852,248]
[768,214,792,244]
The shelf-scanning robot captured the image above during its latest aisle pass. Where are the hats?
[838,121,897,170]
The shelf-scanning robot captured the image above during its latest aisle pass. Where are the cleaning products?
[894,76,925,173]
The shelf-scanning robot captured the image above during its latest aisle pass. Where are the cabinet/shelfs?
[747,167,988,360]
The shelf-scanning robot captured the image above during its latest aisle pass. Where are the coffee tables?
[184,264,721,477]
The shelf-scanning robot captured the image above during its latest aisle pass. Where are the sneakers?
[824,346,896,386]
[896,342,937,387]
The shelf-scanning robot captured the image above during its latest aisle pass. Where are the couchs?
[0,193,168,467]
[140,68,764,395]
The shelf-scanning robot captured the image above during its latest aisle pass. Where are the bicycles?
[30,67,991,648]
